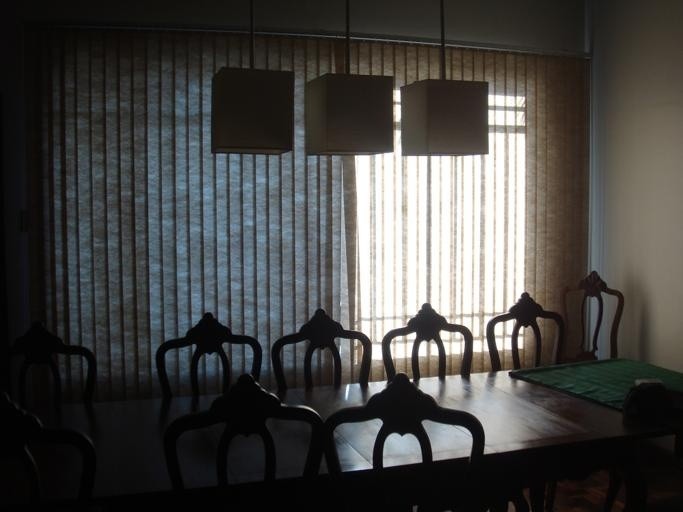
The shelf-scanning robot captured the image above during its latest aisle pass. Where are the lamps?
[398,3,489,157]
[209,2,295,156]
[304,0,394,156]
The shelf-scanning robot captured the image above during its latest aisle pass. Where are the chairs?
[160,372,325,511]
[486,291,571,370]
[562,268,626,367]
[381,303,474,381]
[154,312,262,396]
[271,308,371,388]
[4,318,99,404]
[600,377,682,510]
[1,389,96,511]
[319,372,488,512]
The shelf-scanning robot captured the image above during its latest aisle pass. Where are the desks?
[0,364,681,512]
